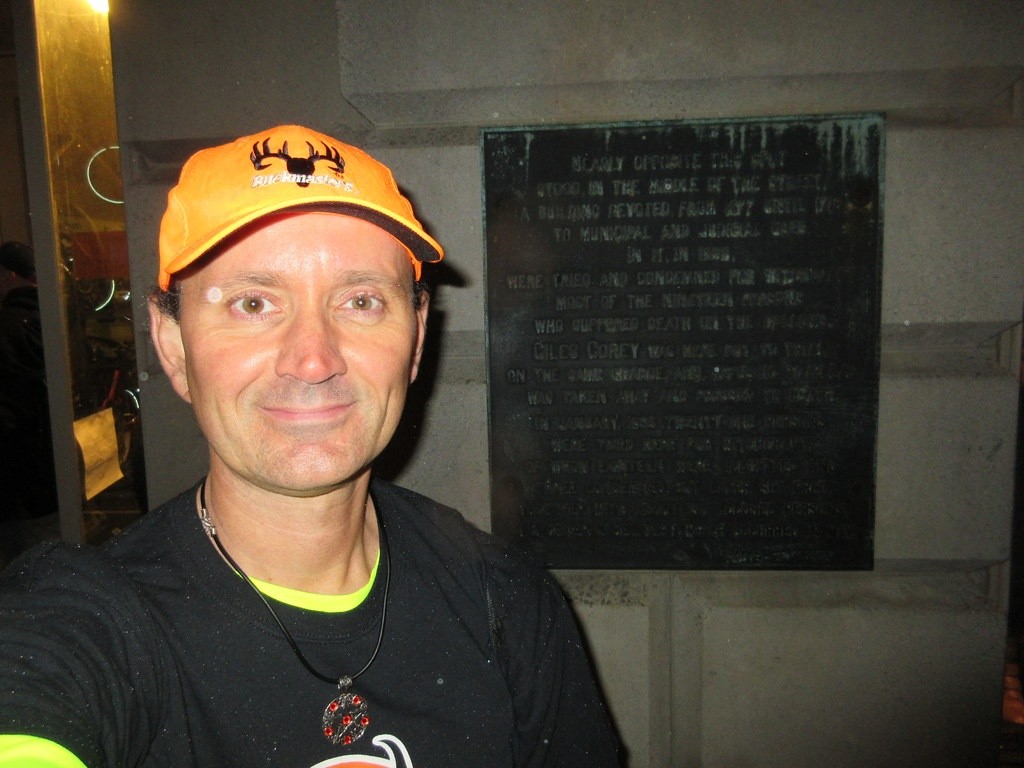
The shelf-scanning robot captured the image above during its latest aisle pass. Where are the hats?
[157,124,444,291]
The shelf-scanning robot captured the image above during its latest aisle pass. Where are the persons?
[0,241,68,515]
[0,124,630,768]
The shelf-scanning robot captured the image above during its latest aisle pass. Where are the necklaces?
[200,476,392,745]
